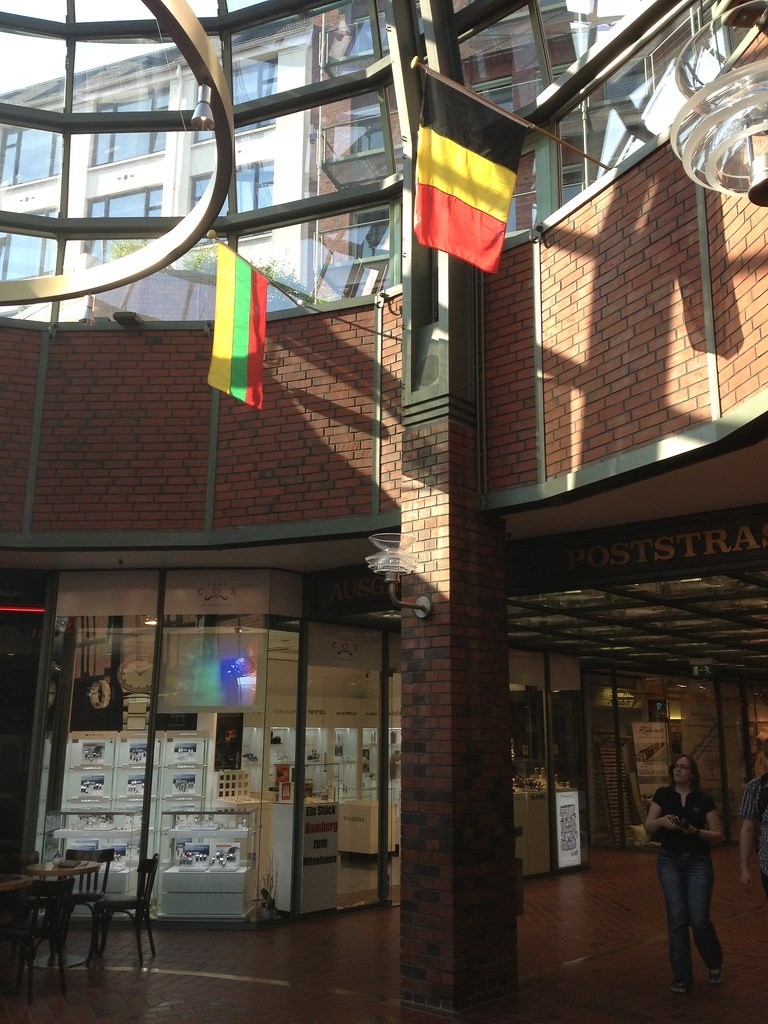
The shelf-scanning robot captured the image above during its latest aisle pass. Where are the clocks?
[116,653,153,693]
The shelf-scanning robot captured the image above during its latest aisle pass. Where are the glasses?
[673,764,691,771]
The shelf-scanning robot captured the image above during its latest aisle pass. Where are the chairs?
[42,847,115,956]
[0,878,76,1006]
[0,851,40,877]
[85,853,160,969]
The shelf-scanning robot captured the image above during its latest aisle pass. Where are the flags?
[206,244,266,409]
[414,71,527,274]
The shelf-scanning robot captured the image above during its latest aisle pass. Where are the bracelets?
[695,828,700,835]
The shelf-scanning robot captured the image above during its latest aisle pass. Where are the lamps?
[364,533,434,619]
[78,293,95,326]
[668,0,768,208]
[190,82,216,131]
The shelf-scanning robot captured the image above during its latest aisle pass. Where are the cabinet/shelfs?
[40,696,403,918]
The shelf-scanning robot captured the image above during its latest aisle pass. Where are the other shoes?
[708,968,722,985]
[670,983,688,993]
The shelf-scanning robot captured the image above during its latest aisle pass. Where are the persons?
[739,742,767,899]
[642,754,726,992]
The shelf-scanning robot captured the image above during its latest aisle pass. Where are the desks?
[26,860,101,970]
[0,873,33,994]
